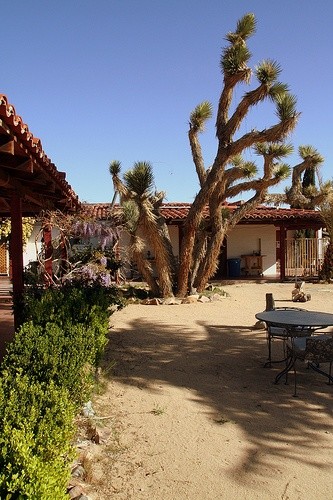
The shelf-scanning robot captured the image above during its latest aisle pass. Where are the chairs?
[251,292,333,398]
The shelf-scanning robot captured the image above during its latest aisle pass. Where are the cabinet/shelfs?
[242,255,266,277]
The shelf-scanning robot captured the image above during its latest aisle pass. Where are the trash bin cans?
[227,258,241,277]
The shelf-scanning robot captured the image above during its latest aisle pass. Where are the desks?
[255,310,333,385]
